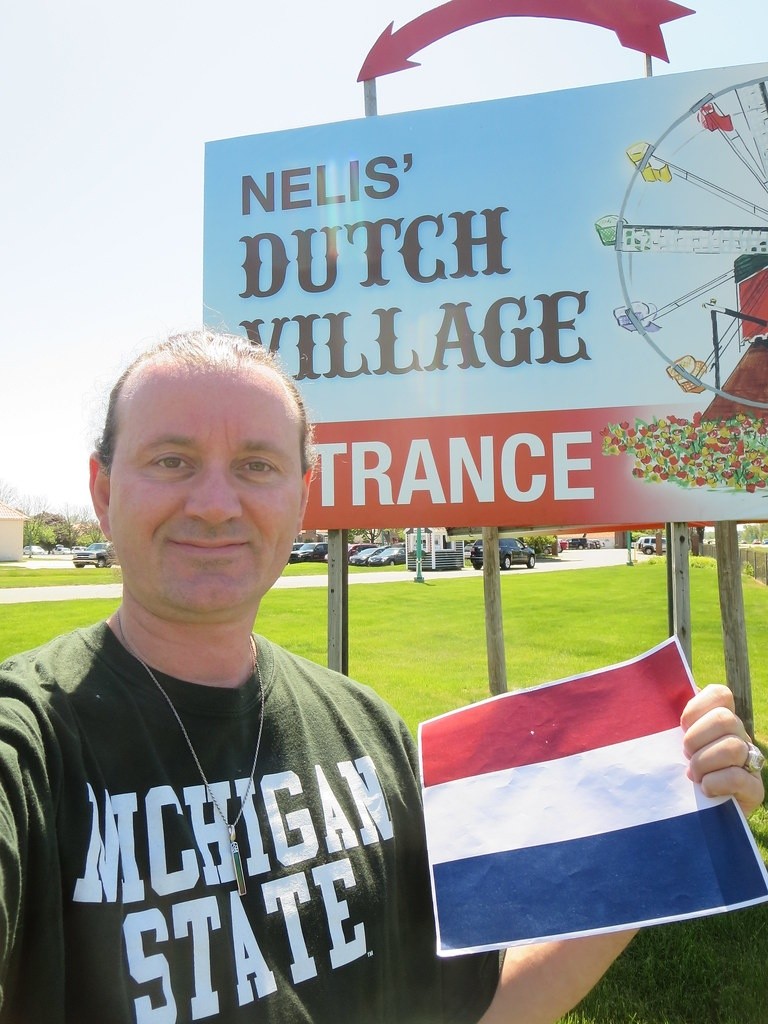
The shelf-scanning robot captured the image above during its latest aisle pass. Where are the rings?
[744,740,765,773]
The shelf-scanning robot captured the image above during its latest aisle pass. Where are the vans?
[324,544,378,563]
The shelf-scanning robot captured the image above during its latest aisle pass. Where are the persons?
[0,331,765,1024]
[583,533,586,537]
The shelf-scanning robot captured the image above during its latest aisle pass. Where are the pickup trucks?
[543,540,568,555]
[637,536,668,555]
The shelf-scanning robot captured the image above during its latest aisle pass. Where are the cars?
[367,548,406,565]
[71,546,85,553]
[348,548,383,565]
[51,544,70,555]
[23,545,44,555]
[588,540,600,549]
[462,543,475,558]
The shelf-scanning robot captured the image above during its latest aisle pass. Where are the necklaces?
[117,609,265,896]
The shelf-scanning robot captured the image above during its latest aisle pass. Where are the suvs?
[286,542,329,564]
[72,543,119,568]
[471,539,536,570]
[569,538,588,549]
